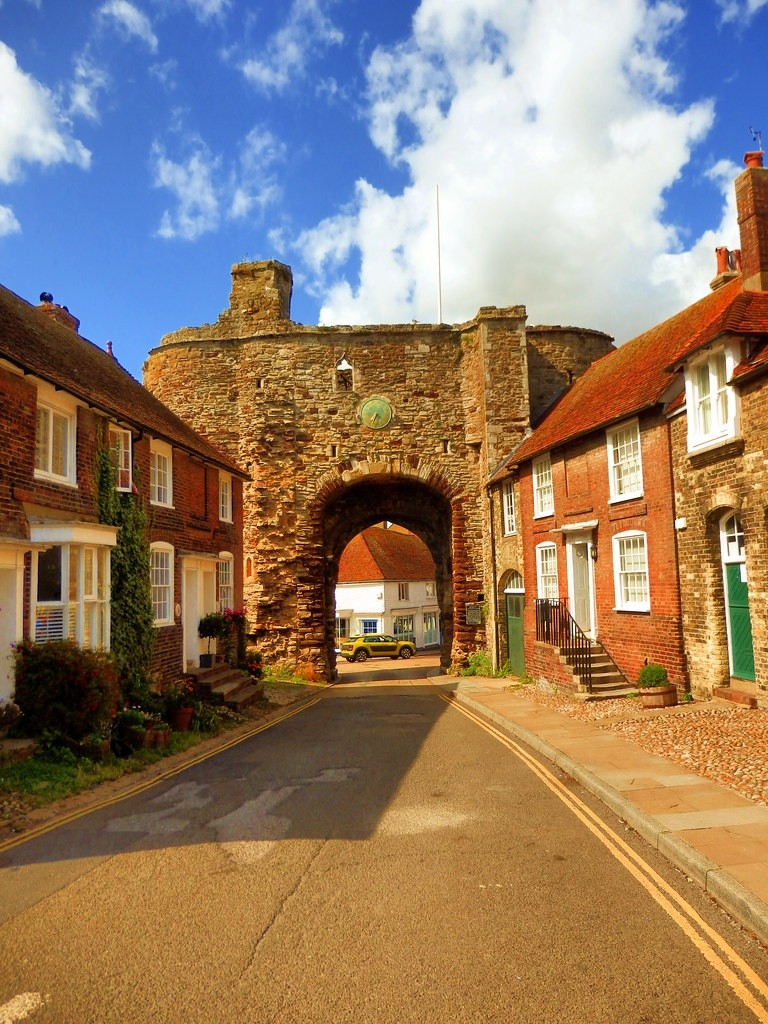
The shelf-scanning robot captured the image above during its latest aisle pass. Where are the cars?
[340,633,416,663]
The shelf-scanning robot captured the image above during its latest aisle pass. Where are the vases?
[171,708,194,731]
[251,679,257,685]
[214,654,226,664]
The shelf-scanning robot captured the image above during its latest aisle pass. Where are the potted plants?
[198,611,230,668]
[637,665,679,708]
[143,712,155,728]
[61,718,113,760]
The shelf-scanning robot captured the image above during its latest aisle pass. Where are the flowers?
[121,705,144,724]
[170,677,195,709]
[245,658,268,679]
[218,608,248,654]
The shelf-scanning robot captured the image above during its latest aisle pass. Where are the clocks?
[359,398,392,431]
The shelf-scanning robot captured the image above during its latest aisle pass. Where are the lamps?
[590,543,598,561]
